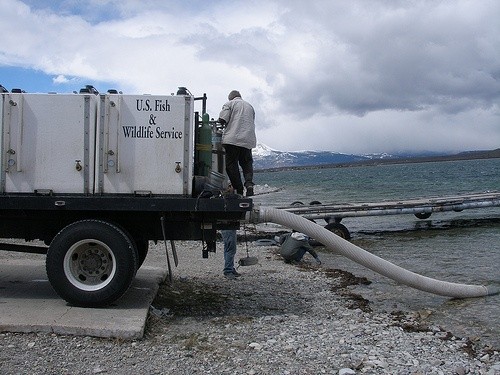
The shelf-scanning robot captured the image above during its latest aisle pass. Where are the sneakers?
[284,259,301,265]
[237,187,243,195]
[222,268,241,280]
[246,186,253,196]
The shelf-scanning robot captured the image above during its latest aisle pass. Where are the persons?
[280,231,322,265]
[218,90,256,196]
[221,230,241,278]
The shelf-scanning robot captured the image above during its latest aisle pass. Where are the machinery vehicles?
[0,84,253,306]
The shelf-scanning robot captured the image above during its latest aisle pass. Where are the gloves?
[315,257,321,265]
